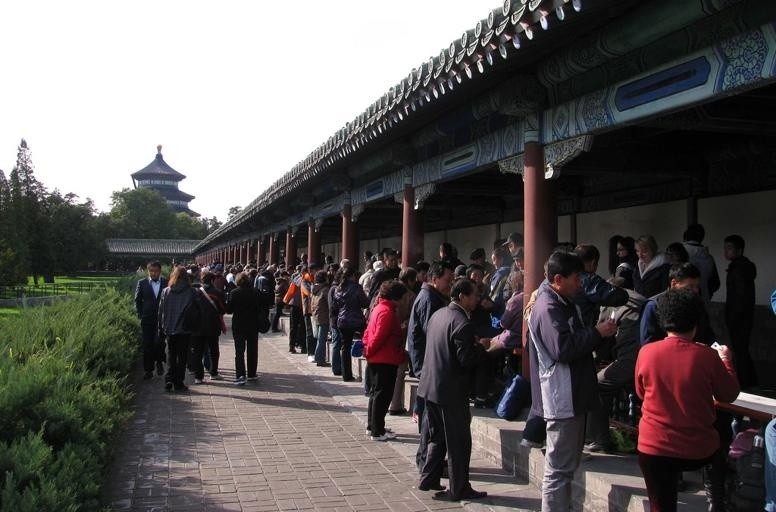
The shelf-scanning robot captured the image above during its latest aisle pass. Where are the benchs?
[709,387,776,426]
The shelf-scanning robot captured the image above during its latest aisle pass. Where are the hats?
[500,232,523,246]
[511,247,523,260]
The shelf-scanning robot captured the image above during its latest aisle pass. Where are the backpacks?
[721,420,766,512]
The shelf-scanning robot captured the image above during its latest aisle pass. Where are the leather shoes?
[475,398,495,408]
[389,407,407,416]
[583,441,608,451]
[449,489,487,501]
[418,484,446,491]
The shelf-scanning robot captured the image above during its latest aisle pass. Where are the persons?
[418,278,502,500]
[134,226,757,446]
[763,417,776,511]
[530,250,616,512]
[407,259,456,471]
[634,289,743,511]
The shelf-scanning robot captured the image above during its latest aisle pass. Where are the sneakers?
[175,386,189,392]
[164,382,172,392]
[247,374,259,381]
[366,428,391,436]
[195,378,202,383]
[210,374,225,380]
[233,375,246,384]
[317,360,330,367]
[371,432,396,440]
[519,438,544,448]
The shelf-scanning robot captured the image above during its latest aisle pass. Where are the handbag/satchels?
[258,308,271,334]
[217,314,227,337]
[495,374,530,420]
